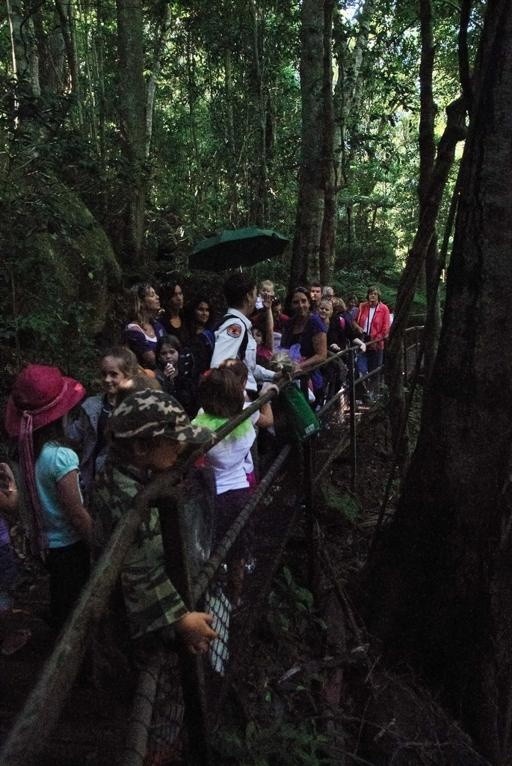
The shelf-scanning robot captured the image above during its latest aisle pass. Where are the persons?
[0,273,394,655]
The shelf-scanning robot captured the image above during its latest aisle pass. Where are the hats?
[111,388,210,445]
[4,365,85,437]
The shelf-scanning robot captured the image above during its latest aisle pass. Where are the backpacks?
[192,315,248,369]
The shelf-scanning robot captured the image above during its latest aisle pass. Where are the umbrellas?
[188,227,290,273]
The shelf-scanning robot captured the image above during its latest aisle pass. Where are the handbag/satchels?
[282,383,321,439]
